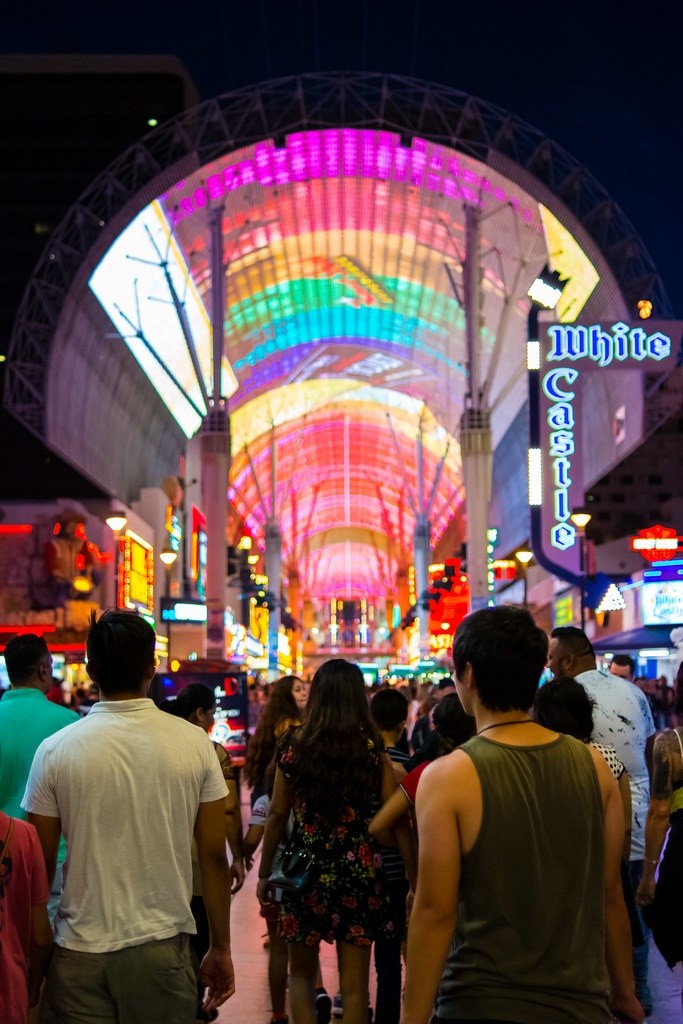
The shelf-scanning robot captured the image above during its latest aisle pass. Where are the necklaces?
[476,720,533,734]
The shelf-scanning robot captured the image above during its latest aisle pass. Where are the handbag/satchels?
[267,846,320,902]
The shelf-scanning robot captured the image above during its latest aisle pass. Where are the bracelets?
[644,856,659,864]
[257,874,267,879]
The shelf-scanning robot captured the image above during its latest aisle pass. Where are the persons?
[243,627,683,1023]
[42,508,104,597]
[0,634,80,1024]
[46,677,99,719]
[406,607,647,1024]
[159,684,244,968]
[19,610,235,1024]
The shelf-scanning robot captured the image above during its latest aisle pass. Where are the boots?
[633,941,654,1016]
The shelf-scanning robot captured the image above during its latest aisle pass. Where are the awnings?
[592,624,683,657]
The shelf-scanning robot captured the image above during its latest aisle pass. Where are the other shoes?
[271,1015,289,1024]
[332,994,343,1016]
[313,987,332,1024]
